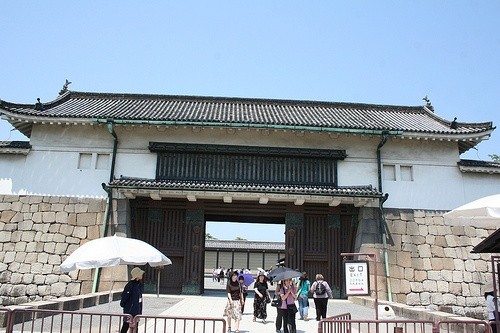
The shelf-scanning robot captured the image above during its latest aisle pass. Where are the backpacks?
[315,280,325,295]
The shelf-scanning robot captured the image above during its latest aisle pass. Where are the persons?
[120,267,145,333]
[484,284,500,333]
[212,266,334,333]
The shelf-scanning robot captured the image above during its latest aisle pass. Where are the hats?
[229,270,238,279]
[131,266,145,280]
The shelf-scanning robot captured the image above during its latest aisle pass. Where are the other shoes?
[316,317,320,321]
[236,329,239,333]
[304,316,307,321]
[253,317,256,322]
[262,321,267,324]
[228,327,231,333]
[276,329,281,333]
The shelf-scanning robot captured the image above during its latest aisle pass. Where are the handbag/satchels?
[271,299,282,307]
[287,304,298,314]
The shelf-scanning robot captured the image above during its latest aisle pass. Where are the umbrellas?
[60,233,172,333]
[237,267,303,289]
[443,194,500,228]
